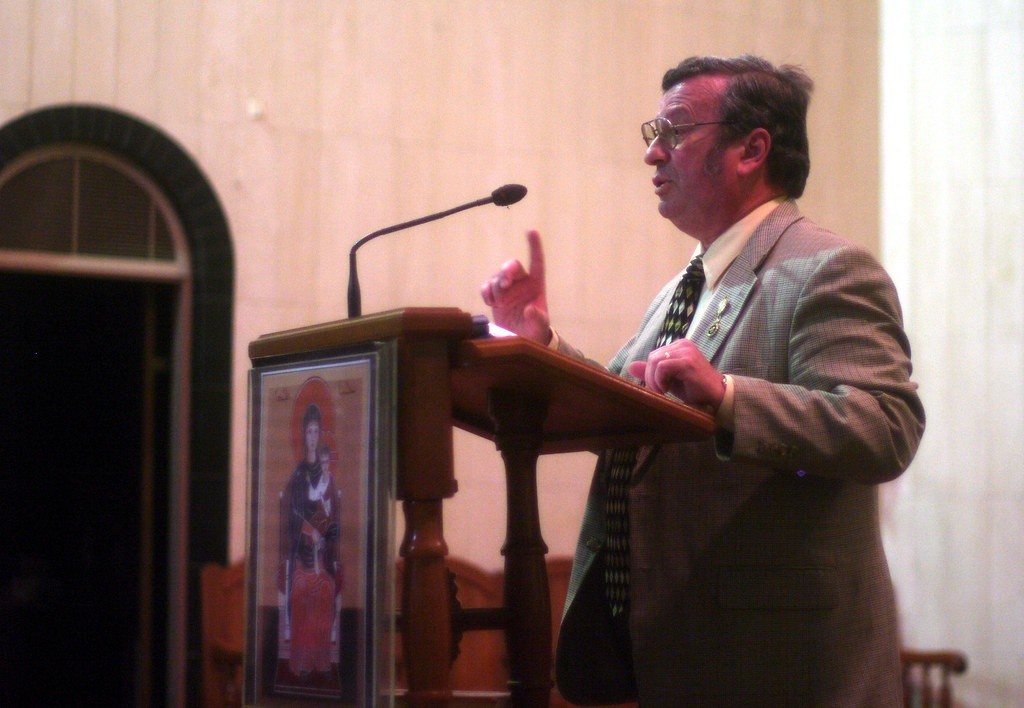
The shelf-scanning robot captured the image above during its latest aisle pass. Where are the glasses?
[641,117,752,152]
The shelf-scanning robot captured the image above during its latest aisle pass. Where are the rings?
[664,352,670,359]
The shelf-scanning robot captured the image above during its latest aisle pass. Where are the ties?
[602,255,705,617]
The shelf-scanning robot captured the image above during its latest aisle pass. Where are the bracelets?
[722,378,727,385]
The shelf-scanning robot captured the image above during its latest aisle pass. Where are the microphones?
[348,182,529,318]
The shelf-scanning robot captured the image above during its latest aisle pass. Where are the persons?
[480,54,925,707]
